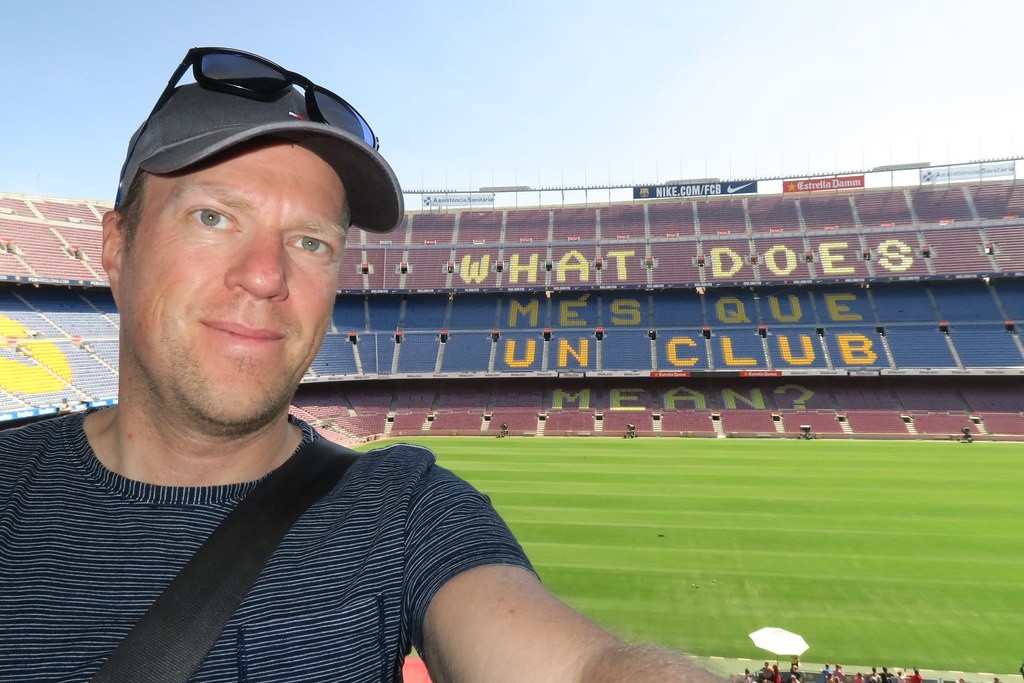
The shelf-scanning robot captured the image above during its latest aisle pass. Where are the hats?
[115,83,405,233]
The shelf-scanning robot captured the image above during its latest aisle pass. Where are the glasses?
[111,47,377,223]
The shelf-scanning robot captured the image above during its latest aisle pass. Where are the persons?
[0,79,735,683]
[733,656,1024,683]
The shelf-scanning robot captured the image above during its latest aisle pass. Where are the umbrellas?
[748,627,809,668]
